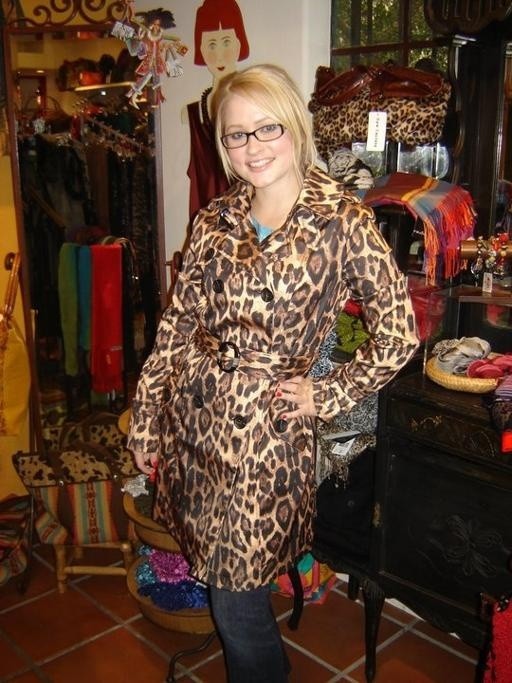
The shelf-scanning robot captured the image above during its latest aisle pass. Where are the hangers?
[83,115,156,163]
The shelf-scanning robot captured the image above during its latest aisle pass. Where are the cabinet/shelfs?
[359,40,512,272]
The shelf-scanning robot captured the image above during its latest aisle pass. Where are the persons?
[22,168,88,337]
[112,4,174,110]
[126,63,421,683]
[180,0,249,219]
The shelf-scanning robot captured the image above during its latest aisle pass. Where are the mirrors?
[1,1,167,451]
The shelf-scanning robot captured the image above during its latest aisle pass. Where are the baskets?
[425,352,501,395]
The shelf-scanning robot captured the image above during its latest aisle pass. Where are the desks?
[287,370,512,683]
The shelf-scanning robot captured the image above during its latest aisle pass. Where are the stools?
[54,542,131,593]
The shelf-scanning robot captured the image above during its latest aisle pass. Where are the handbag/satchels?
[41,412,130,450]
[11,446,142,544]
[308,66,453,158]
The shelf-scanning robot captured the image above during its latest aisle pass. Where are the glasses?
[219,123,285,148]
